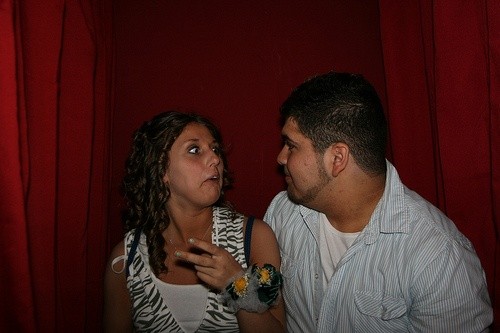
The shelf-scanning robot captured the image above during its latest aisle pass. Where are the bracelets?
[219,262,287,314]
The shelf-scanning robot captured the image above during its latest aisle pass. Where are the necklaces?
[166,217,214,253]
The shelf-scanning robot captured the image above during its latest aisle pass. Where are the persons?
[103,109,288,332]
[260,69,494,333]
